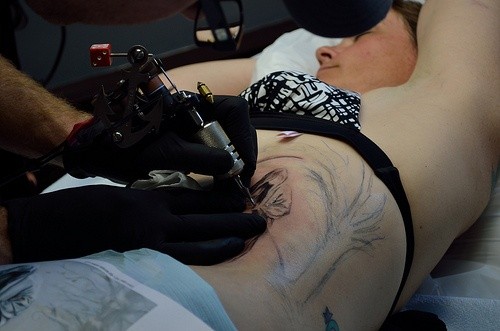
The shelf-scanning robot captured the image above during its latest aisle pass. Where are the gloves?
[54,89,256,188]
[4,183,268,279]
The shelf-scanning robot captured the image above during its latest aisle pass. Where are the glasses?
[192,1,244,50]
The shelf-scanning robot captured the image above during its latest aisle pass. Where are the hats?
[285,1,395,38]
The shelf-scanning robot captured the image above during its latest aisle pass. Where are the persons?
[0,52,266,266]
[0,0,500,331]
